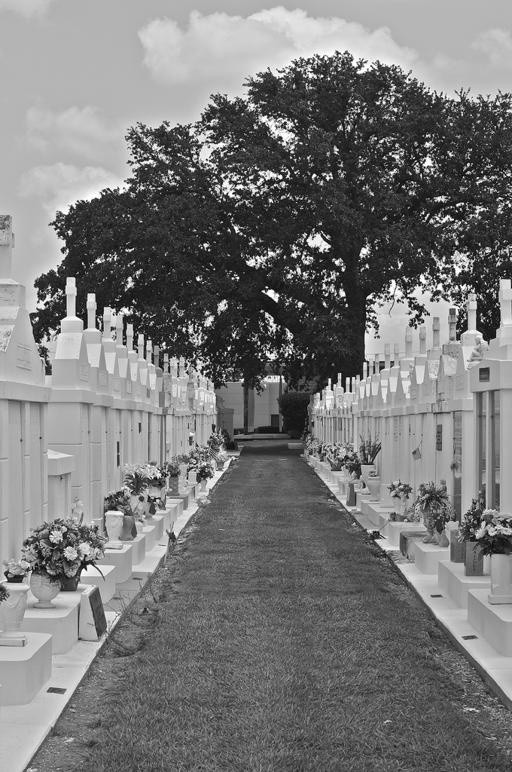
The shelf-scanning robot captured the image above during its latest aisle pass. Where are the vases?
[30,571,63,608]
[60,571,81,592]
[2,582,30,648]
[302,448,512,606]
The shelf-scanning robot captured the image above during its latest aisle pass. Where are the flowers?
[102,448,225,550]
[1,431,229,602]
[302,433,512,557]
[2,512,109,584]
